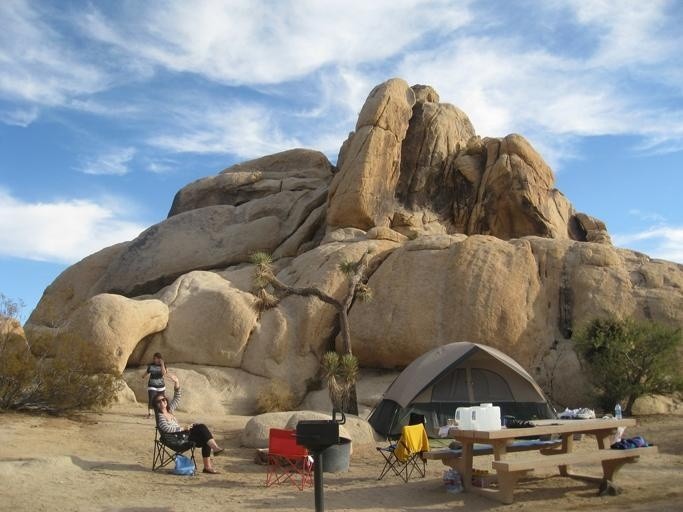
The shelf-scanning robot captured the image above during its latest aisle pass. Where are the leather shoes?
[203,469,221,474]
[213,449,225,456]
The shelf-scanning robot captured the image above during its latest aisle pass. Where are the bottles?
[472,468,490,488]
[442,468,462,493]
[614,402,622,420]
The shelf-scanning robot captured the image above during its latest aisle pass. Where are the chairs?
[266,428,315,491]
[152,420,198,472]
[376,422,425,483]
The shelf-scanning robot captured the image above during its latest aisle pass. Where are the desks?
[448,418,636,505]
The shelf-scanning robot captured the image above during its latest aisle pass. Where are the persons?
[140,350,167,418]
[152,371,226,475]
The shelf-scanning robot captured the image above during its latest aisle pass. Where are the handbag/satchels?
[175,453,195,475]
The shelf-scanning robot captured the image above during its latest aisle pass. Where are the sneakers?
[556,407,595,419]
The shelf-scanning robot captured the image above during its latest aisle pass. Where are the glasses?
[156,398,166,403]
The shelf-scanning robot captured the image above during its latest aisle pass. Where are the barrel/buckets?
[454,402,502,433]
[322,436,353,474]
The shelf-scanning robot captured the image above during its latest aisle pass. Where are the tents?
[364,341,561,447]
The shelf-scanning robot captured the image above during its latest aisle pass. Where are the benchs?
[422,439,658,505]
